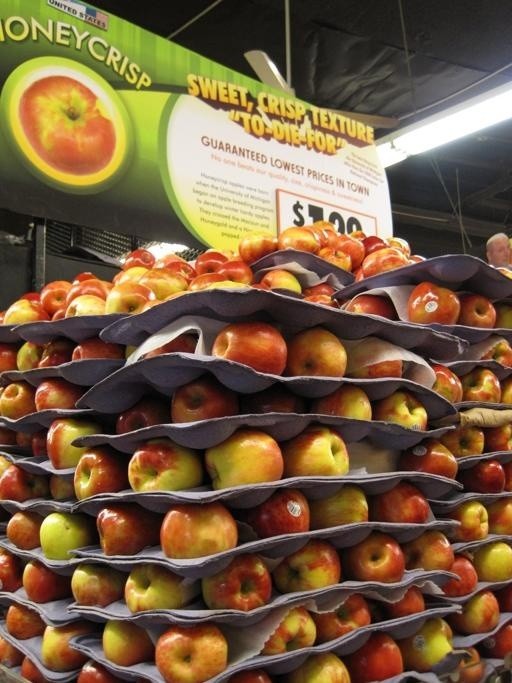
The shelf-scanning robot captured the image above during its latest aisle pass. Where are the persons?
[484,231,511,268]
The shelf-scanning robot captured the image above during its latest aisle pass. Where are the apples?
[18,77,115,176]
[0,223,512,683]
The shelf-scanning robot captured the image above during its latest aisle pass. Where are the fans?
[244,2,400,133]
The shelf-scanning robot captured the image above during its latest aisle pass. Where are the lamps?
[374,2,512,171]
